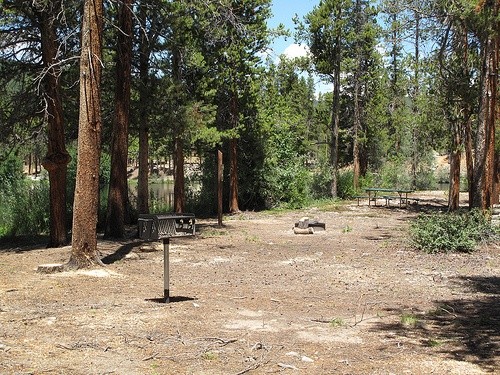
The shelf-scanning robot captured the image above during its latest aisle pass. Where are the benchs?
[352,194,383,207]
[384,195,420,208]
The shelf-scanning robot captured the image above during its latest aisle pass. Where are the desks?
[365,187,413,209]
